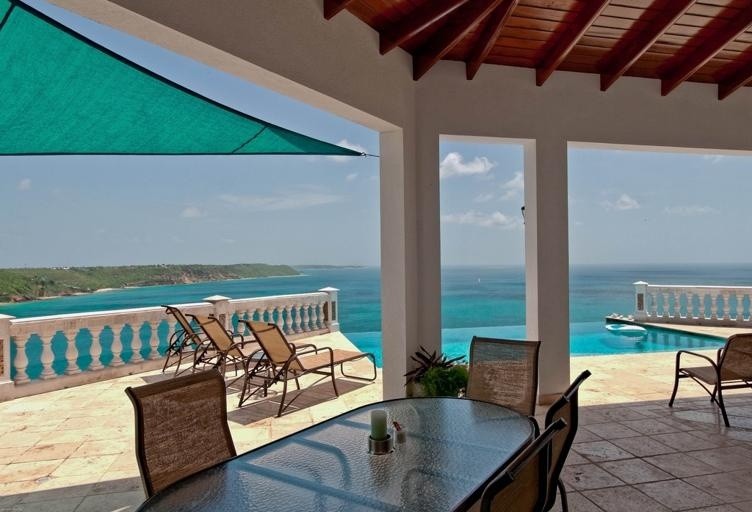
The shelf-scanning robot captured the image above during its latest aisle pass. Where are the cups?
[394,429,407,442]
[367,434,395,455]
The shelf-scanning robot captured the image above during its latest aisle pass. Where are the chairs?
[466,415,568,512]
[545,369,592,512]
[668,332,752,428]
[159,304,378,416]
[124,367,239,502]
[465,335,541,420]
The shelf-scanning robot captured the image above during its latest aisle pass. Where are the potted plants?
[398,340,471,401]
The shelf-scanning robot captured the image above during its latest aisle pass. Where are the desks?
[137,396,539,511]
